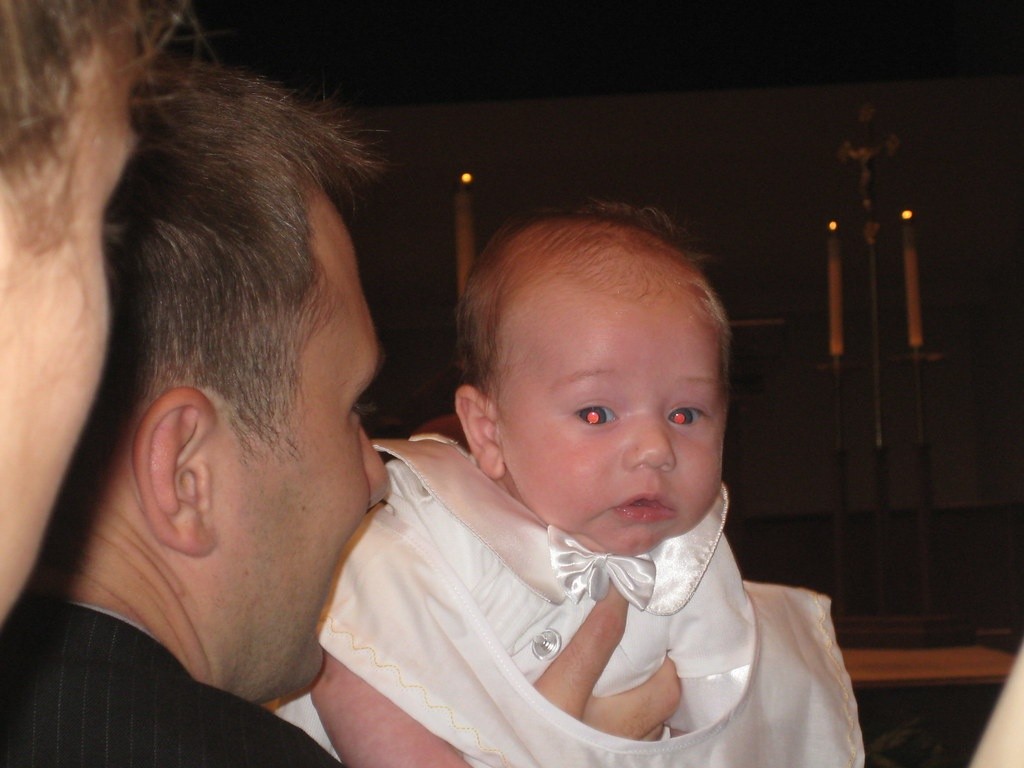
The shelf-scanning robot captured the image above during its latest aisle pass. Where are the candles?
[901,205,923,349]
[456,170,479,301]
[826,219,850,356]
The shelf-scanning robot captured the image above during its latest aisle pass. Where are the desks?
[843,644,1013,689]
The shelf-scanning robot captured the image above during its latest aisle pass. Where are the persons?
[275,198,864,768]
[0,62,395,768]
[0,0,180,630]
